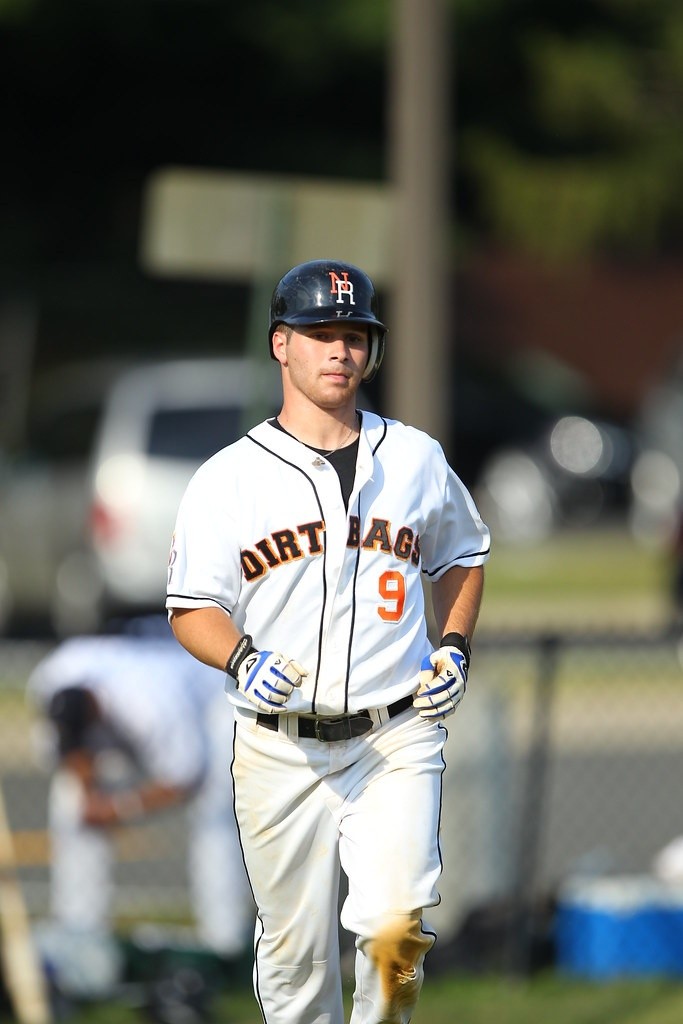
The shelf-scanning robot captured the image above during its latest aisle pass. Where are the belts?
[234,693,419,742]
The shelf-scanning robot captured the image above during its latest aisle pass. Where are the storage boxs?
[550,901,682,981]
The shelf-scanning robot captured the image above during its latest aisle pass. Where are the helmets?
[268,259,386,384]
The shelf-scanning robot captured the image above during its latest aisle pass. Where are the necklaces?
[282,413,359,467]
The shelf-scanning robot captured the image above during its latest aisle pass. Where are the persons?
[15,632,253,1003]
[159,262,492,1024]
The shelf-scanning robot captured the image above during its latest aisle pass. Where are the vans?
[87,354,285,635]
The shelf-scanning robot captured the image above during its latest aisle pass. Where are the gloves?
[226,634,305,713]
[415,633,471,724]
